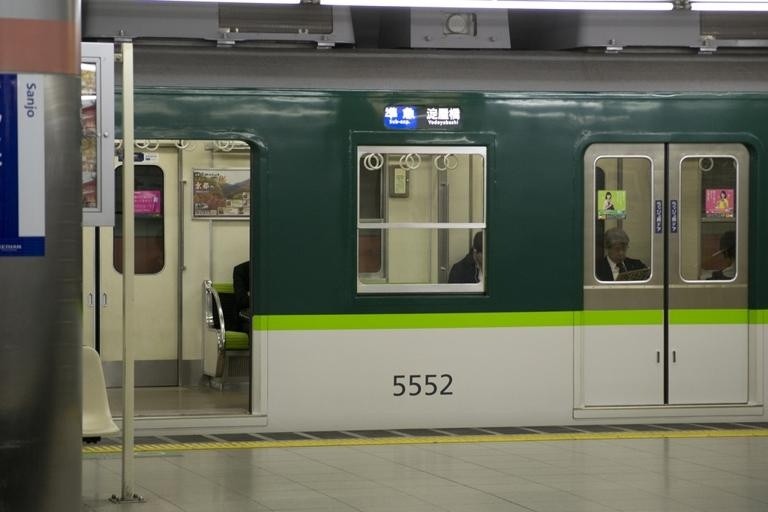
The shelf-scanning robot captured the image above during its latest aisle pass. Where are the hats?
[712,230,736,260]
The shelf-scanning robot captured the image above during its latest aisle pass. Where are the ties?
[616,262,626,273]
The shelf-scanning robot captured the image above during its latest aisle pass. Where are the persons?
[232,261,251,334]
[602,192,616,218]
[448,230,483,284]
[596,228,651,280]
[715,191,732,213]
[704,230,737,279]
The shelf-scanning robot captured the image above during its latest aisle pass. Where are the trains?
[81,38,768,433]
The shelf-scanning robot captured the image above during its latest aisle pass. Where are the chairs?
[79,344,120,437]
[200,280,251,387]
